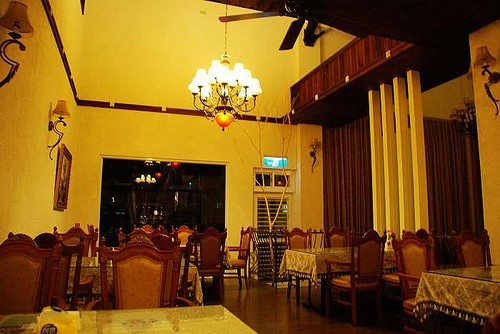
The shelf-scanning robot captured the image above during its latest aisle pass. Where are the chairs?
[0,223,500,334]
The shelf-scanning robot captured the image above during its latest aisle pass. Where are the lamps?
[473,46,500,116]
[450,96,477,141]
[48,100,70,160]
[309,137,322,173]
[135,174,157,184]
[189,5,262,132]
[0,0,34,88]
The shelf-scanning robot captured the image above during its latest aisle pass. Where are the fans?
[218,0,370,50]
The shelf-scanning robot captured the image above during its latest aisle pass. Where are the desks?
[412,265,500,334]
[0,303,257,334]
[278,246,399,318]
[179,244,232,270]
[67,256,203,304]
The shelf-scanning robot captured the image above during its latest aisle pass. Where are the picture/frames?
[53,143,72,209]
